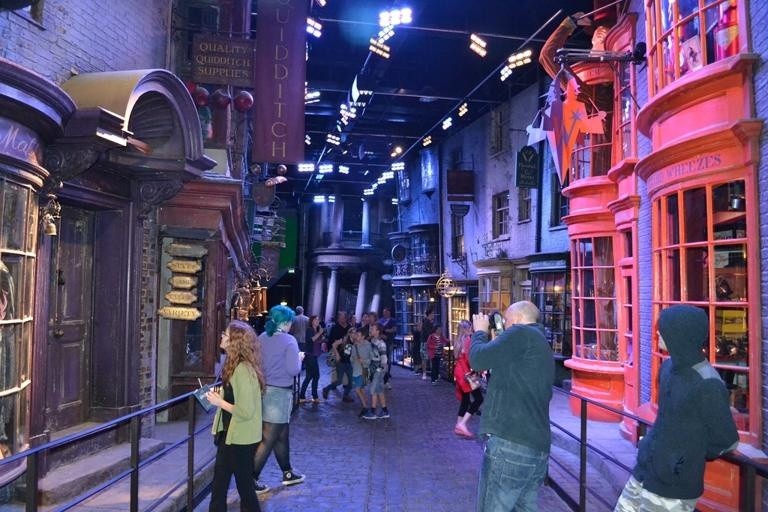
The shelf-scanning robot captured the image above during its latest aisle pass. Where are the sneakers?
[323,387,328,399]
[343,396,352,402]
[385,383,391,390]
[360,408,390,420]
[454,423,474,436]
[414,369,440,384]
[312,398,320,402]
[253,479,270,493]
[282,470,305,485]
[300,398,307,402]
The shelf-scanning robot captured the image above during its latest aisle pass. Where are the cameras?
[488,312,504,333]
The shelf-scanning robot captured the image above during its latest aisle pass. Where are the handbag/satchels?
[327,349,337,367]
[465,371,481,390]
[214,431,223,445]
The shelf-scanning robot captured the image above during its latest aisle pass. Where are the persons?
[425,323,450,385]
[449,320,485,438]
[410,322,422,376]
[466,299,556,512]
[250,304,307,498]
[205,320,264,512]
[417,307,442,382]
[613,304,741,511]
[290,306,398,421]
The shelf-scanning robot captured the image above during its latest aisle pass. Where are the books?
[193,383,217,413]
